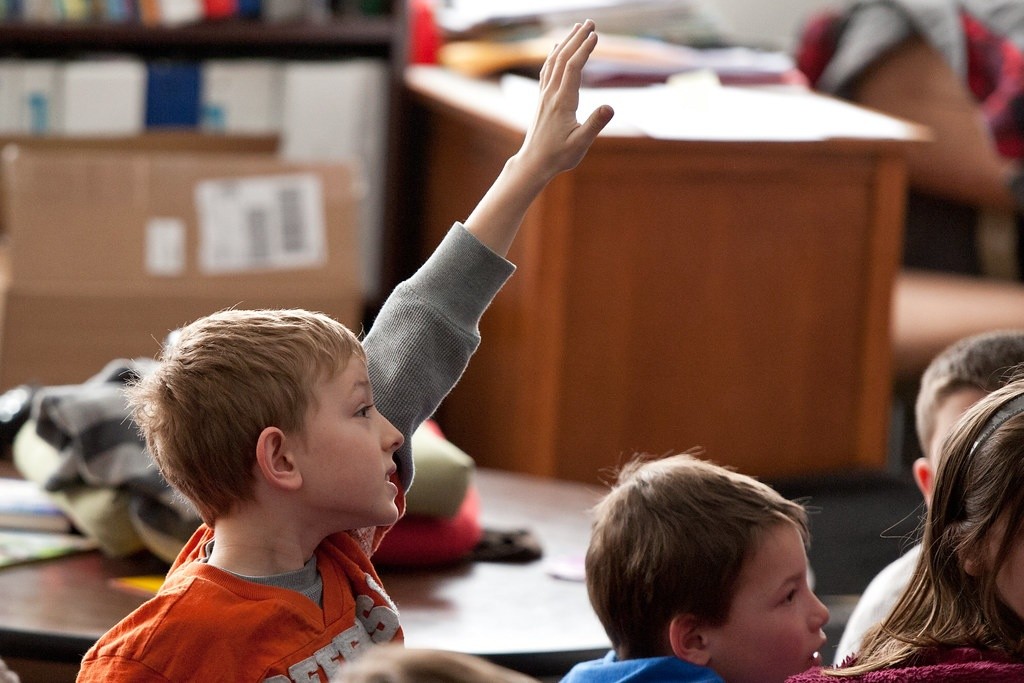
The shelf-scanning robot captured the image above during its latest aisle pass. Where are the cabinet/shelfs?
[0,1,407,407]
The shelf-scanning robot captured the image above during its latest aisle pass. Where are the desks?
[407,58,940,487]
[1,469,654,683]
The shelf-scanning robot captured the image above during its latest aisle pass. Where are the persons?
[783,381,1024,683]
[834,329,1024,669]
[558,456,830,683]
[72,18,615,683]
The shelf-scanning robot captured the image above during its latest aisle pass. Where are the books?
[0,478,73,534]
